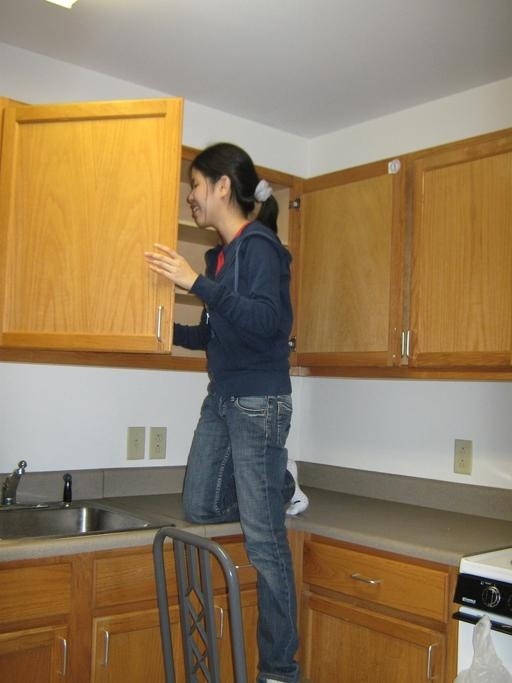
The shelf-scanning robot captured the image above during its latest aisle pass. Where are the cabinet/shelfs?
[0,97,512,377]
[191,542,262,682]
[294,535,459,681]
[0,542,190,681]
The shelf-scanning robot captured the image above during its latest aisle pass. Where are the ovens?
[451,574,512,683]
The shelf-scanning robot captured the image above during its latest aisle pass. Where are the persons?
[145,141,310,683]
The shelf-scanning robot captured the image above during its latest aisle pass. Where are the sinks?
[0,501,176,543]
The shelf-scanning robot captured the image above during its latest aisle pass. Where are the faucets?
[2,460,28,504]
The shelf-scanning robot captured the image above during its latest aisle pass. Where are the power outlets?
[148,425,167,459]
[453,438,473,475]
